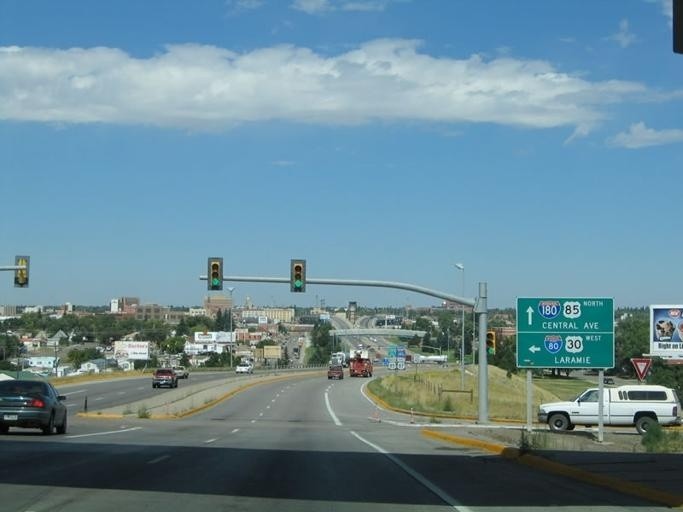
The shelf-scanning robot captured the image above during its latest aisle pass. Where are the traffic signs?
[515,296,615,369]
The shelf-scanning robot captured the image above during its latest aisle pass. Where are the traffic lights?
[16,256,29,286]
[210,262,220,291]
[487,331,495,355]
[294,264,304,292]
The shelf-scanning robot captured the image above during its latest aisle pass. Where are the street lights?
[455,263,465,389]
[228,287,233,365]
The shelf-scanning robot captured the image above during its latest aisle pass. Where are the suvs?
[538,384,682,435]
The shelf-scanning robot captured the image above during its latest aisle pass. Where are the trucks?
[328,347,377,379]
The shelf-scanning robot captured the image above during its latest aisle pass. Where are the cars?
[0,379,68,432]
[173,366,189,378]
[152,368,178,388]
[236,364,253,374]
